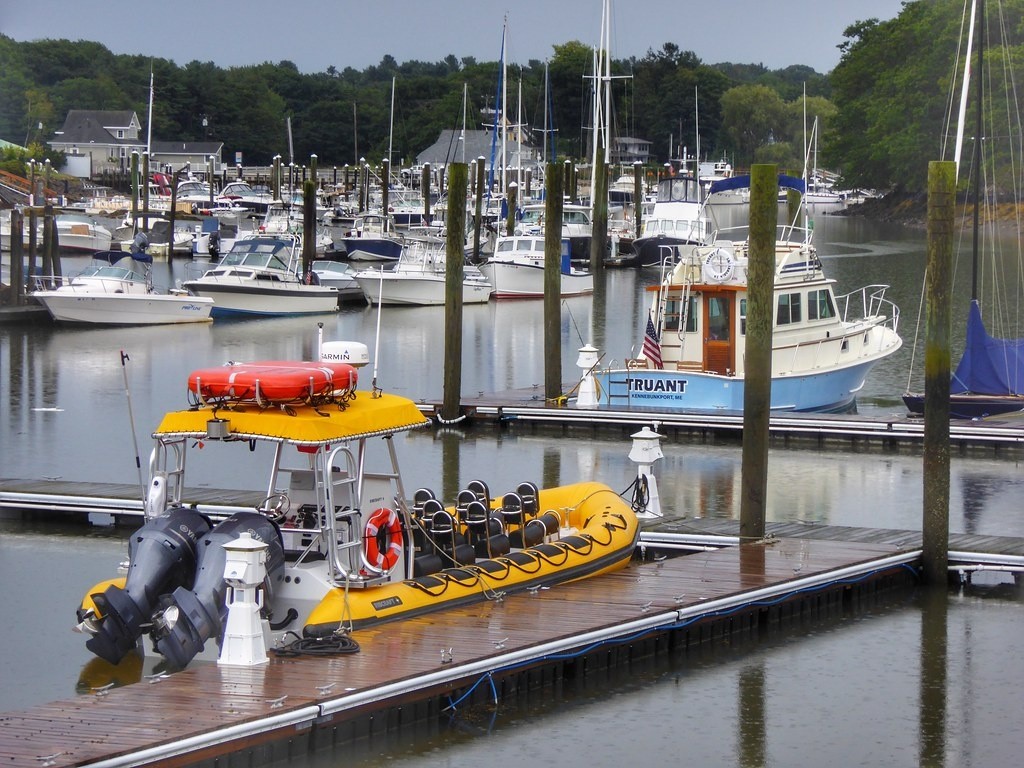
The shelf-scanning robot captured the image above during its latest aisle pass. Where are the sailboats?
[902,1,1024,420]
[0,0,872,324]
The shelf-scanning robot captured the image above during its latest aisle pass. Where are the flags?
[642,313,663,369]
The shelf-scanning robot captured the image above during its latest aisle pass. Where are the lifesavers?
[389,207,394,212]
[363,508,402,571]
[310,272,319,285]
[704,249,734,281]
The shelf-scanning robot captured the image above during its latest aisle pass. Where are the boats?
[72,267,667,669]
[575,79,904,416]
